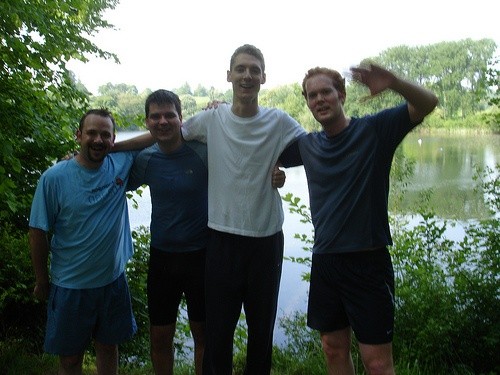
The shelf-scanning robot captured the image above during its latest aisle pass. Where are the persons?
[64,44,308,375]
[202,59,439,375]
[27,108,142,375]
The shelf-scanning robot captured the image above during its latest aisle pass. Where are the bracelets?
[34,277,50,286]
